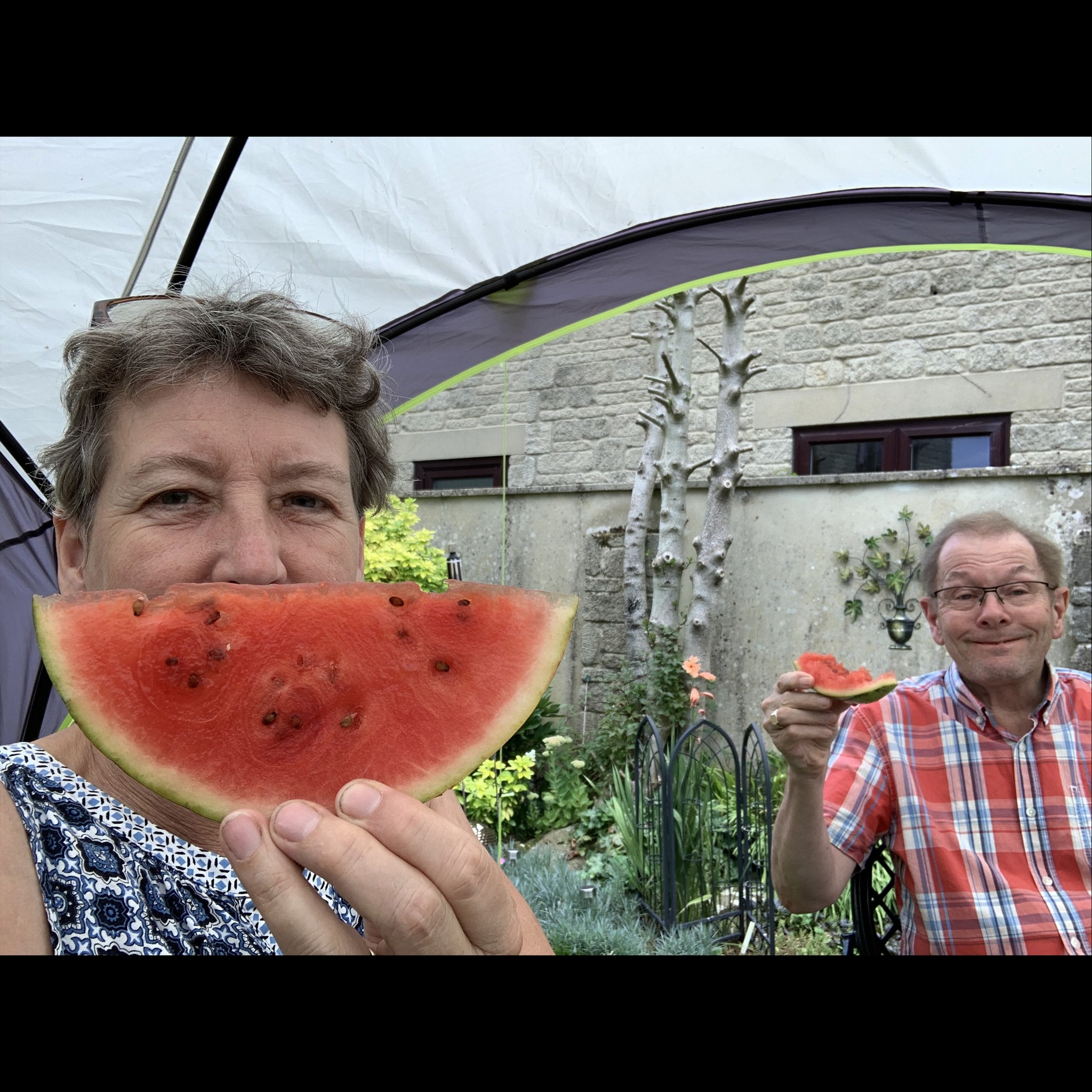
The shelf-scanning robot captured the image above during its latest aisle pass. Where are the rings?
[770,709,783,729]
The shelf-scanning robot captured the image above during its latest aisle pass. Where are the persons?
[1,287,555,957]
[762,512,1091,957]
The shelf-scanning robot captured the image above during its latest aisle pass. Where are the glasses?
[90,295,358,350]
[932,580,1054,608]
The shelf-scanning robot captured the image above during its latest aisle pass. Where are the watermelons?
[33,578,580,823]
[793,652,897,704]
